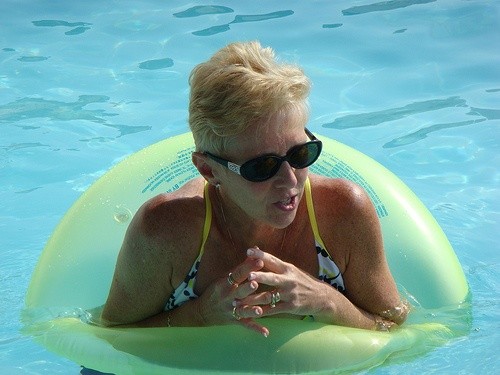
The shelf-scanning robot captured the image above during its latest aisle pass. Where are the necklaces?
[219,197,286,255]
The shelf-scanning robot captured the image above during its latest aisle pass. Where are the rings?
[270,292,281,307]
[227,273,239,288]
[232,307,241,321]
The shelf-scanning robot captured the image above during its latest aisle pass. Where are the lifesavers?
[20,130,473,375]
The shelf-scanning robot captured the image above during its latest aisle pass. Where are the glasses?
[202,126,323,182]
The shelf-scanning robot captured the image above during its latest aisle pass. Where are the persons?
[95,42,408,339]
